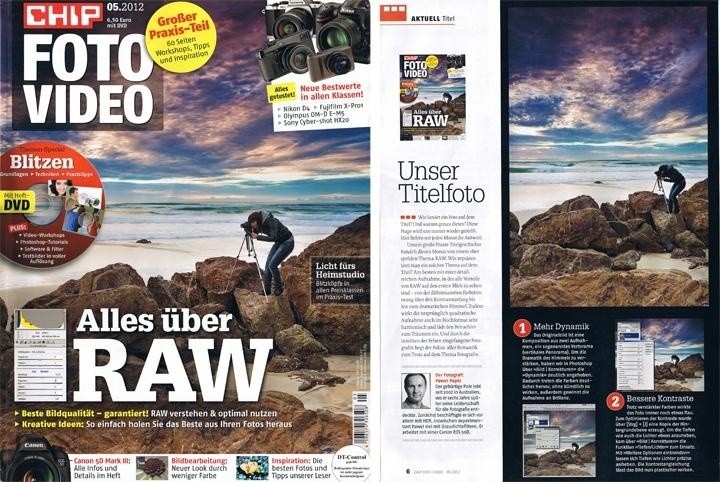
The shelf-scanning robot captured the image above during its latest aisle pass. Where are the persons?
[671,353,681,366]
[402,372,431,410]
[49,178,102,237]
[245,207,297,298]
[442,91,453,103]
[656,163,687,215]
[572,441,580,454]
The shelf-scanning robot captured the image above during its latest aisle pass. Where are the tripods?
[225,233,272,305]
[652,177,671,215]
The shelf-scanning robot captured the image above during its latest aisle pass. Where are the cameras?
[240,221,251,232]
[0,431,70,482]
[655,171,663,176]
[256,0,370,83]
